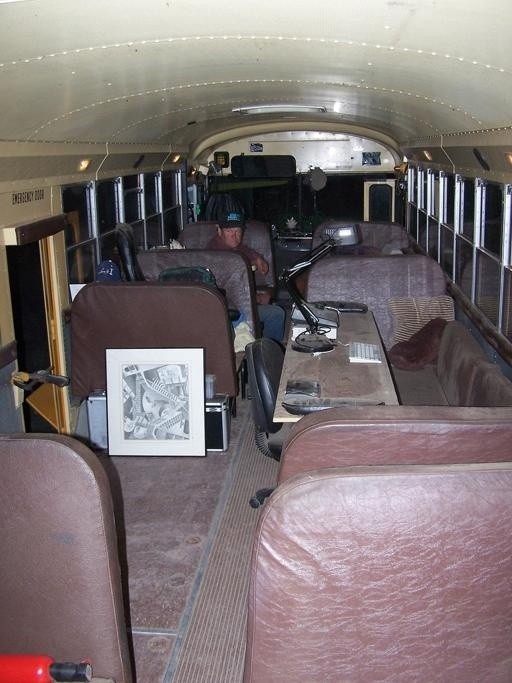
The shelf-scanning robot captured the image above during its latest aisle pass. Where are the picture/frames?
[106,347,205,458]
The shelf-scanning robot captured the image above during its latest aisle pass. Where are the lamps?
[277,221,362,355]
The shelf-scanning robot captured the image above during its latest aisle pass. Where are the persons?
[205,211,287,342]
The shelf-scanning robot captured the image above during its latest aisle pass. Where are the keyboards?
[348,341,382,363]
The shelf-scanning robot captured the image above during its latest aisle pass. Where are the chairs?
[242,462,512,681]
[274,406,512,490]
[244,336,294,507]
[3,431,137,681]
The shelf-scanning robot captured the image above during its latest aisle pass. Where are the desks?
[272,310,399,434]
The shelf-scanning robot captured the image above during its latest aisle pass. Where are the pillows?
[388,318,448,372]
[389,295,455,343]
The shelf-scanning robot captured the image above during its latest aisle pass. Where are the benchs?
[70,280,247,418]
[178,222,278,303]
[311,220,410,256]
[386,321,512,410]
[135,249,261,344]
[306,255,447,344]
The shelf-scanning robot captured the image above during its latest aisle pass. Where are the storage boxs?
[86,388,232,454]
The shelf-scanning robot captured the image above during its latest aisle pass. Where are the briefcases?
[87,391,107,449]
[205,392,231,451]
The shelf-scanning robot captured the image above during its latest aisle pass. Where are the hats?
[96,260,121,281]
[220,212,247,228]
